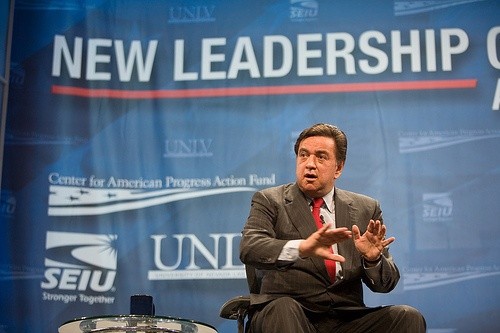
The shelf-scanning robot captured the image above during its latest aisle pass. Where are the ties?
[310,199,336,280]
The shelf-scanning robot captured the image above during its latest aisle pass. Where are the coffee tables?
[56,315,218,333]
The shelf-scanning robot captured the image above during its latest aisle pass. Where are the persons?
[238,123,428,333]
